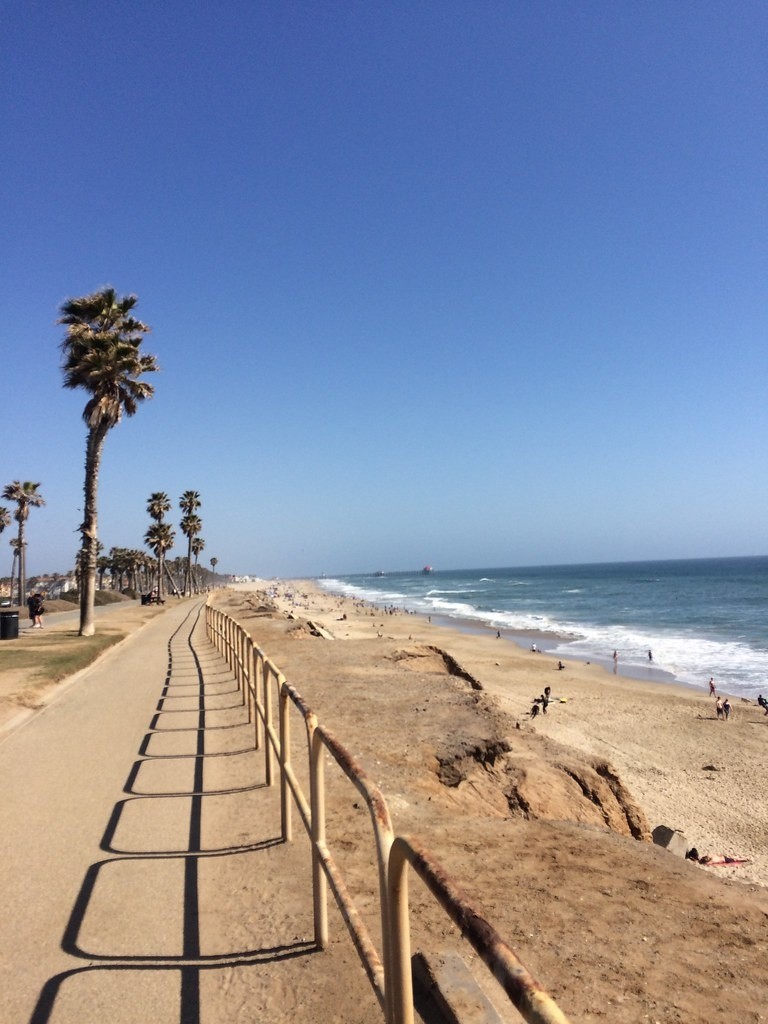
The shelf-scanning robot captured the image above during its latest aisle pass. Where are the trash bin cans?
[0,610,20,641]
[141,595,150,605]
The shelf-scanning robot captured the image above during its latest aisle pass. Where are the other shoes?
[33,624,37,628]
[40,625,44,629]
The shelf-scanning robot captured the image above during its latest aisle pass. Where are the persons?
[147,586,210,606]
[261,583,768,720]
[27,591,45,628]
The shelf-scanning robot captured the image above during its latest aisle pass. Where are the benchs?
[147,597,166,606]
